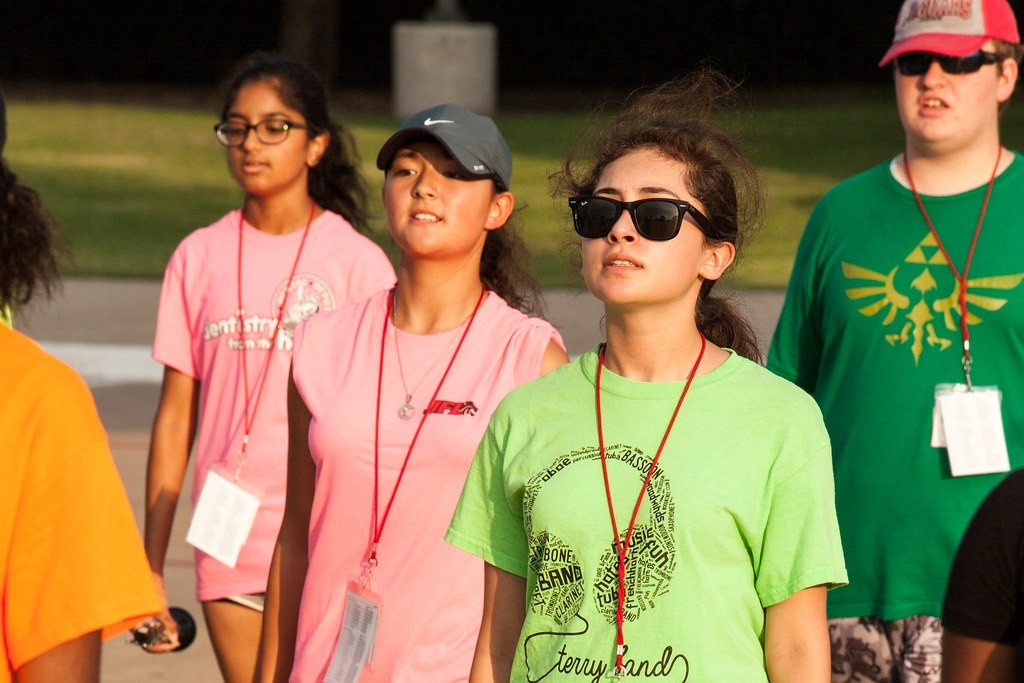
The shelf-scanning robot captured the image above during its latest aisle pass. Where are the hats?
[376,103,512,190]
[879,0,1020,66]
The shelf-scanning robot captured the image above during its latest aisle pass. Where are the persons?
[134,70,849,683]
[767,1,1023,681]
[1,163,163,683]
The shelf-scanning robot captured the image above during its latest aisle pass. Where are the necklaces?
[393,282,472,417]
[599,329,706,662]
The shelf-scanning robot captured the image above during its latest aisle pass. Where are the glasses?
[896,48,1001,75]
[215,118,306,147]
[568,195,708,241]
